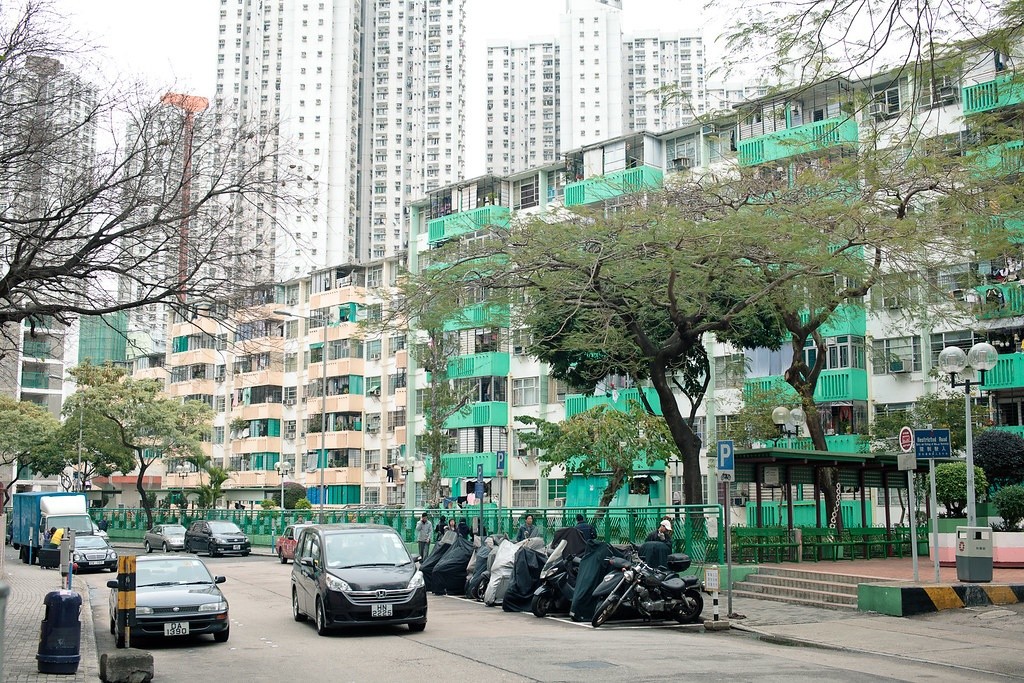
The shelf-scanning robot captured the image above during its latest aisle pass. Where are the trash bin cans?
[39,549,60,569]
[38,590,84,674]
[956,527,993,582]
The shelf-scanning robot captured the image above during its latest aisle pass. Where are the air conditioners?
[380,182,385,185]
[379,246,384,249]
[370,353,379,360]
[367,280,379,288]
[889,361,912,373]
[555,500,565,506]
[940,87,957,100]
[673,159,689,169]
[370,389,380,396]
[869,103,887,117]
[380,198,385,201]
[370,429,378,433]
[366,464,377,470]
[882,296,900,308]
[517,449,531,456]
[702,123,719,137]
[215,299,296,473]
[513,346,528,355]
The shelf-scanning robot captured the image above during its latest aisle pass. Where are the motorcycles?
[592,548,711,629]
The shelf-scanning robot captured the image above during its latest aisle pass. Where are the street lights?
[274,461,292,510]
[273,310,328,515]
[397,456,415,510]
[48,375,83,492]
[772,407,807,562]
[937,341,998,528]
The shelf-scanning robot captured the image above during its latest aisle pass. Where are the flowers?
[846,426,852,432]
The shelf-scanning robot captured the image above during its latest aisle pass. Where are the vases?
[847,431,850,435]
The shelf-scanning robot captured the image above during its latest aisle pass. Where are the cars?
[275,521,319,565]
[291,523,427,636]
[183,520,252,558]
[106,552,229,649]
[142,524,187,554]
[90,520,109,541]
[73,536,118,573]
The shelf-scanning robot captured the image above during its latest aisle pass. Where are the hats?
[660,520,672,530]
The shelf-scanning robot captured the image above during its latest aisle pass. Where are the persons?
[41,527,70,569]
[645,520,673,549]
[99,516,107,532]
[443,519,458,536]
[457,517,470,539]
[574,514,597,541]
[517,514,538,542]
[470,519,486,542]
[295,515,304,524]
[435,516,448,540]
[416,513,432,563]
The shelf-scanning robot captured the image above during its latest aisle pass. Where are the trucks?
[13,491,93,565]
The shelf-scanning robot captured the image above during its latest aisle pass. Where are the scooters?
[419,525,672,618]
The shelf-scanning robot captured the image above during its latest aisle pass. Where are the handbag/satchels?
[437,531,443,540]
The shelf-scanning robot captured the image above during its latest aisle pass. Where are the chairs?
[328,538,345,564]
[136,569,152,585]
[175,565,199,581]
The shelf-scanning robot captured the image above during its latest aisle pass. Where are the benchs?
[801,528,857,563]
[849,528,906,560]
[896,527,929,558]
[735,528,799,565]
